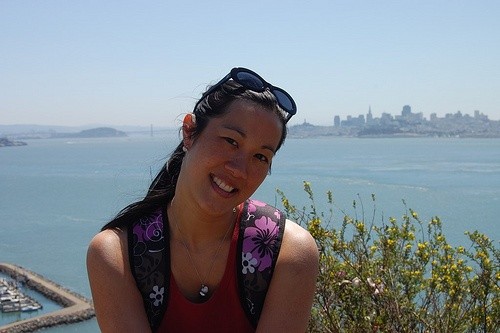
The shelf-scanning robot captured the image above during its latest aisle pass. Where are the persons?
[86,69,319,333]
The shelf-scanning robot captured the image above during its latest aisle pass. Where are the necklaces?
[171,203,234,298]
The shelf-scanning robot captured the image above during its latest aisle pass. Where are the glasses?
[193,67,297,125]
